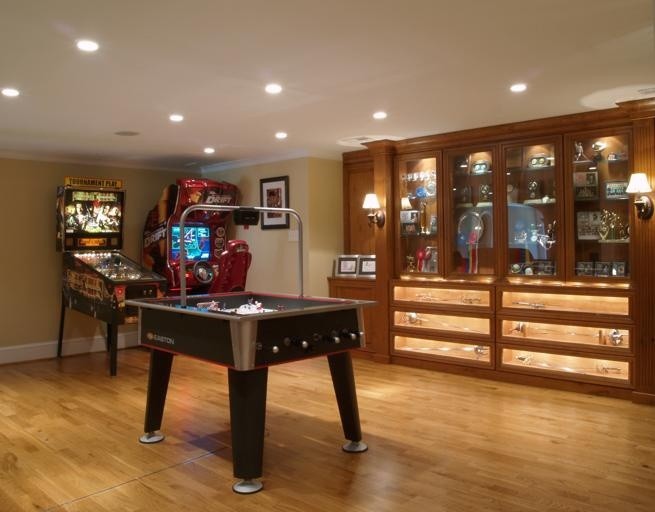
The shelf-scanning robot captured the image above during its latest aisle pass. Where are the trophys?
[397,139,630,277]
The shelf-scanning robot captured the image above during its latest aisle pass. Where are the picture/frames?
[604,180,628,200]
[575,169,600,202]
[259,175,290,229]
[575,209,604,241]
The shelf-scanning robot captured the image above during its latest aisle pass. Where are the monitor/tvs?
[171,221,212,263]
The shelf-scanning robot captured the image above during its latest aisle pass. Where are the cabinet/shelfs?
[391,282,496,371]
[328,278,376,361]
[493,284,632,391]
[391,144,498,282]
[500,123,632,281]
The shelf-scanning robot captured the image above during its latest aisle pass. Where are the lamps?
[626,172,653,220]
[362,194,385,228]
